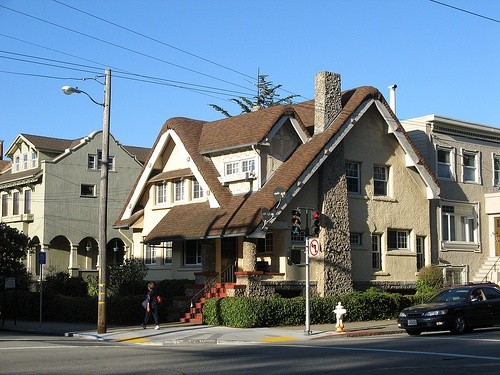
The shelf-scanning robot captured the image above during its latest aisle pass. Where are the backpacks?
[148,291,160,311]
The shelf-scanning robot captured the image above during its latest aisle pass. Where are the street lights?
[62,68,112,333]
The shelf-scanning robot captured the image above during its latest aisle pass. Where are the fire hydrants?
[333,301,347,333]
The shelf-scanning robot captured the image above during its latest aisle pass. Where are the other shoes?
[139,323,146,329]
[154,324,161,330]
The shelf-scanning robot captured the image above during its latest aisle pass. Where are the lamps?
[113,242,119,252]
[86,240,92,252]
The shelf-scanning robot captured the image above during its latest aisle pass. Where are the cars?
[395,282,500,336]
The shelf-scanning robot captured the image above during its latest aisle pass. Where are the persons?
[470,294,481,301]
[139,282,160,330]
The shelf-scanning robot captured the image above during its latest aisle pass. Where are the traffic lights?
[291,208,303,236]
[308,210,320,236]
